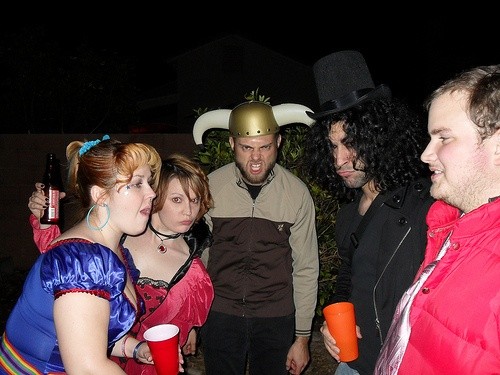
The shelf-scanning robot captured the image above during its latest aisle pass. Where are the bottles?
[39,154,59,225]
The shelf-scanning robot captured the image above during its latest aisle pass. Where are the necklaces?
[148,214,181,254]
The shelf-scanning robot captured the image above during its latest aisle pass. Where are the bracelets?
[122,334,132,358]
[133,341,146,364]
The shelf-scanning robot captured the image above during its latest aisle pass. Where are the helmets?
[229,87,280,138]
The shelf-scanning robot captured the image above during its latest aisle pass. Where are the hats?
[304,49,392,121]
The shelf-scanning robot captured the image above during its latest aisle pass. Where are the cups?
[143,323,180,375]
[323,302,359,362]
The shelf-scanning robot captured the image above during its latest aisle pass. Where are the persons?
[313,49,436,375]
[194,101,319,375]
[0,134,184,375]
[28,154,214,375]
[373,66,500,375]
